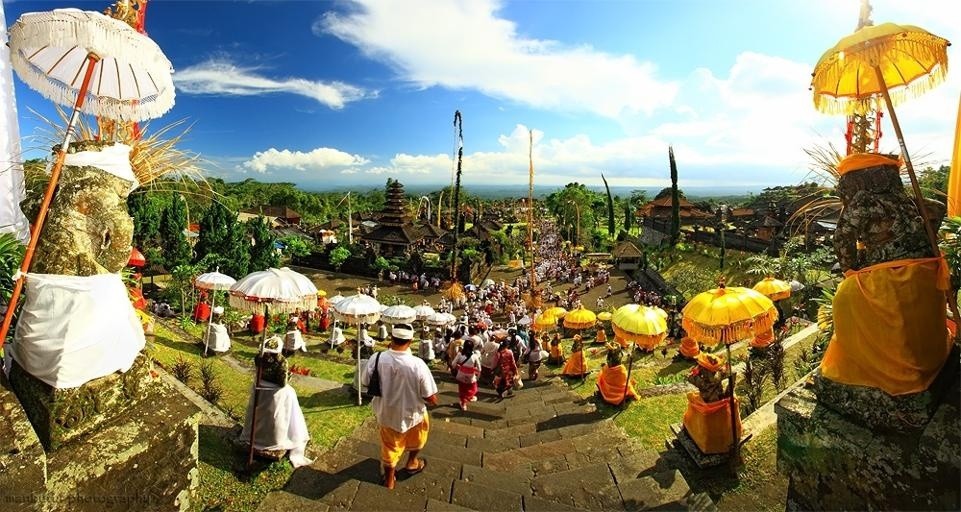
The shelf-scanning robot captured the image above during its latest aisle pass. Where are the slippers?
[406,457,427,475]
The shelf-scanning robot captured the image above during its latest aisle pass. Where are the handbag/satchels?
[513,374,523,389]
[368,351,381,396]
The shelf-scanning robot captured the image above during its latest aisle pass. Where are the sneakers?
[460,401,467,410]
[470,395,477,401]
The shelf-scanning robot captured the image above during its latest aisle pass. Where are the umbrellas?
[809,22,960,350]
[125,245,145,271]
[0,6,179,360]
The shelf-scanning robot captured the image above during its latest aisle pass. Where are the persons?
[420,281,561,411]
[361,322,438,486]
[357,268,441,296]
[243,289,344,468]
[597,283,705,405]
[517,219,611,375]
[156,292,231,356]
